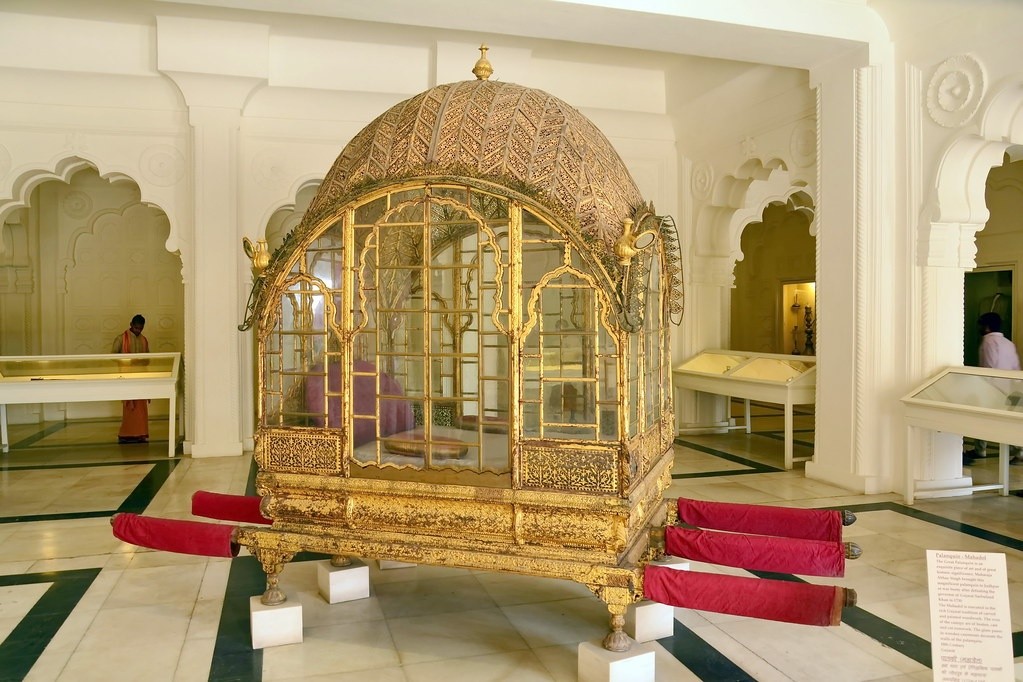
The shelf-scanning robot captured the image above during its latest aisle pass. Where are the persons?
[963,312,1023,466]
[111,315,151,443]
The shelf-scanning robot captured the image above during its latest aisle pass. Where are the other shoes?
[119,437,146,443]
[967,449,986,458]
[1010,456,1023,464]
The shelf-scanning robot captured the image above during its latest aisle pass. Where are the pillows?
[385,434,468,459]
[305,361,415,448]
[454,415,509,434]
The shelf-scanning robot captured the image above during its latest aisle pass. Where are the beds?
[237,43,685,567]
